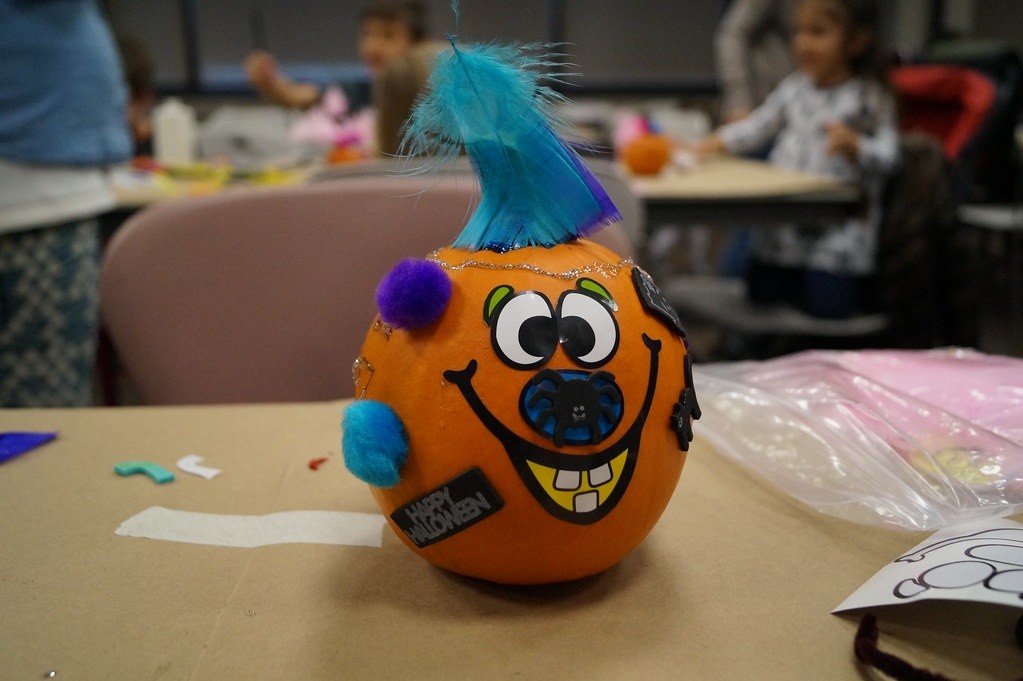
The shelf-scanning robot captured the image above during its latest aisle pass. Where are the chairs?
[99,177,482,405]
[663,62,1002,365]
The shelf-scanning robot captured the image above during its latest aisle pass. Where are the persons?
[242,0,473,158]
[688,0,901,319]
[0,0,158,408]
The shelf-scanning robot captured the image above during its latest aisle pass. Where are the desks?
[0,398,1023,681]
[614,152,856,348]
